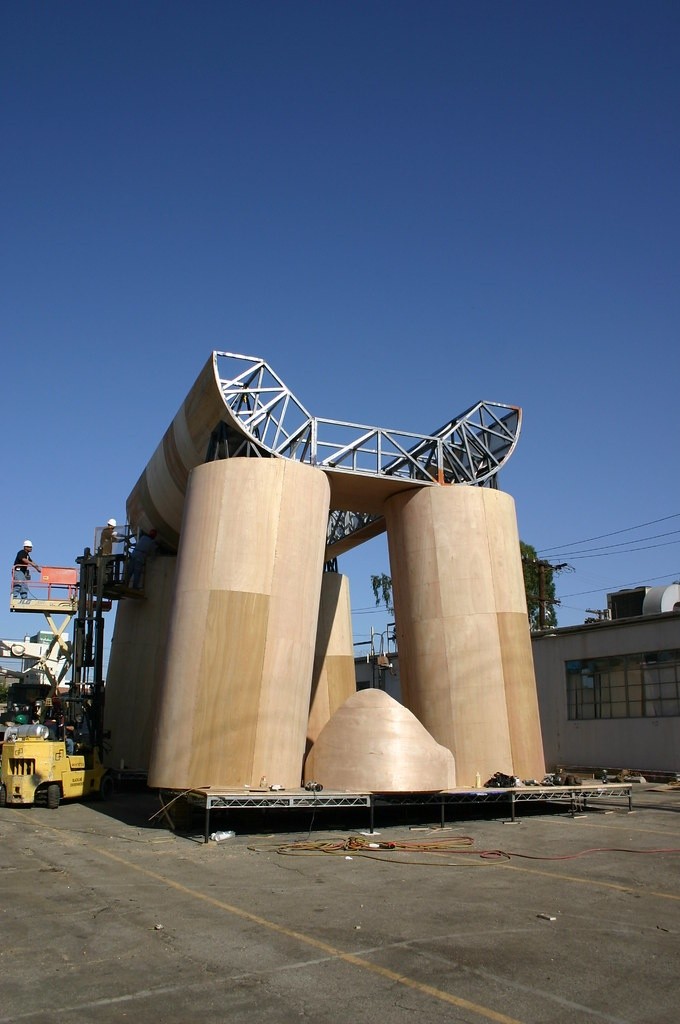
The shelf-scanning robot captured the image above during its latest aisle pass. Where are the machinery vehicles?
[0,697,117,810]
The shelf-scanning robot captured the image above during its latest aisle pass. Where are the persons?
[121,529,158,591]
[99,519,124,582]
[12,540,41,599]
[55,719,75,756]
[7,715,27,738]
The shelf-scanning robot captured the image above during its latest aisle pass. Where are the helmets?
[149,529,157,537]
[23,540,33,547]
[15,715,28,724]
[108,519,117,526]
